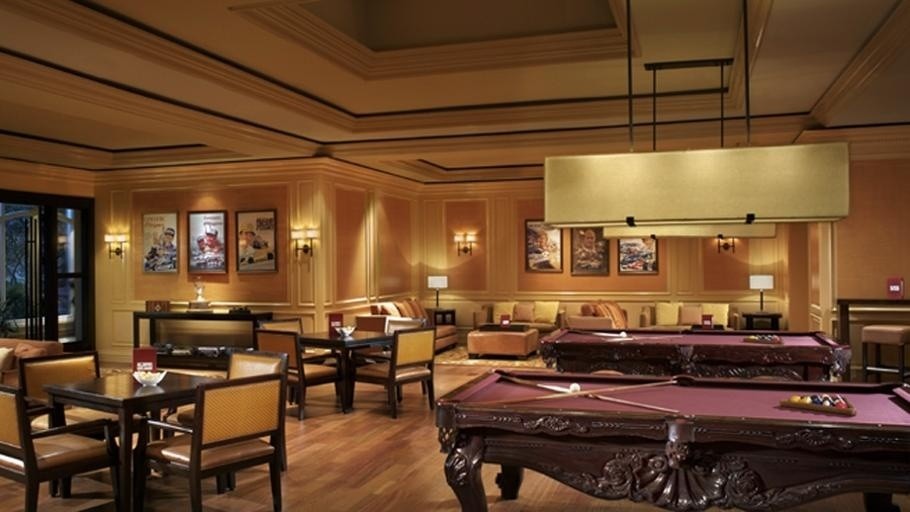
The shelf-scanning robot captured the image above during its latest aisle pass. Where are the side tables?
[435,307,456,324]
[743,309,782,329]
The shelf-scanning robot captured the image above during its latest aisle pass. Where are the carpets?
[433,345,555,369]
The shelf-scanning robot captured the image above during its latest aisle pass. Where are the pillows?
[370,298,432,318]
[0,347,15,375]
[593,303,729,329]
[14,344,47,369]
[493,301,559,324]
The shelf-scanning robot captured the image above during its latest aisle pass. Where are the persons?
[638,236,656,271]
[194,236,207,255]
[203,227,225,264]
[575,228,603,271]
[155,227,176,266]
[237,223,273,266]
[536,232,548,253]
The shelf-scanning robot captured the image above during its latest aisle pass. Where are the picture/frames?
[570,228,609,277]
[187,208,228,273]
[235,208,277,274]
[140,210,178,272]
[525,218,563,272]
[617,238,659,275]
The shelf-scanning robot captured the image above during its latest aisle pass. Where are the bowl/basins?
[334,325,357,336]
[133,387,165,398]
[131,368,168,386]
[337,336,354,342]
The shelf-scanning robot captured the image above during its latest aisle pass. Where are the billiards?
[749,334,779,341]
[569,383,581,393]
[789,392,848,408]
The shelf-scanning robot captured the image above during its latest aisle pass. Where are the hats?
[197,236,204,242]
[206,229,219,235]
[165,227,175,236]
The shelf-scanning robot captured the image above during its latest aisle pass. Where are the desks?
[435,368,910,512]
[538,327,851,381]
[41,370,237,512]
[133,310,273,370]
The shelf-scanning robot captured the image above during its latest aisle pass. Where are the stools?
[861,324,910,384]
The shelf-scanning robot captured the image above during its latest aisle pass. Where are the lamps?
[600,55,779,240]
[454,233,472,256]
[105,233,130,258]
[289,225,318,256]
[544,1,850,225]
[749,275,773,311]
[428,276,448,307]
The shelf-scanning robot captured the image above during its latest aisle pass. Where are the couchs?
[473,302,566,337]
[356,312,457,357]
[568,302,743,331]
[0,337,63,388]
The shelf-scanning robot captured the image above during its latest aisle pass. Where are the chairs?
[1,384,123,510]
[256,314,435,421]
[179,349,289,473]
[16,350,149,500]
[133,372,289,512]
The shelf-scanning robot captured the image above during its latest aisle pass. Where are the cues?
[504,375,679,414]
[458,381,678,406]
[567,329,683,343]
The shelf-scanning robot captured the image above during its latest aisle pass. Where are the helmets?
[239,223,257,236]
